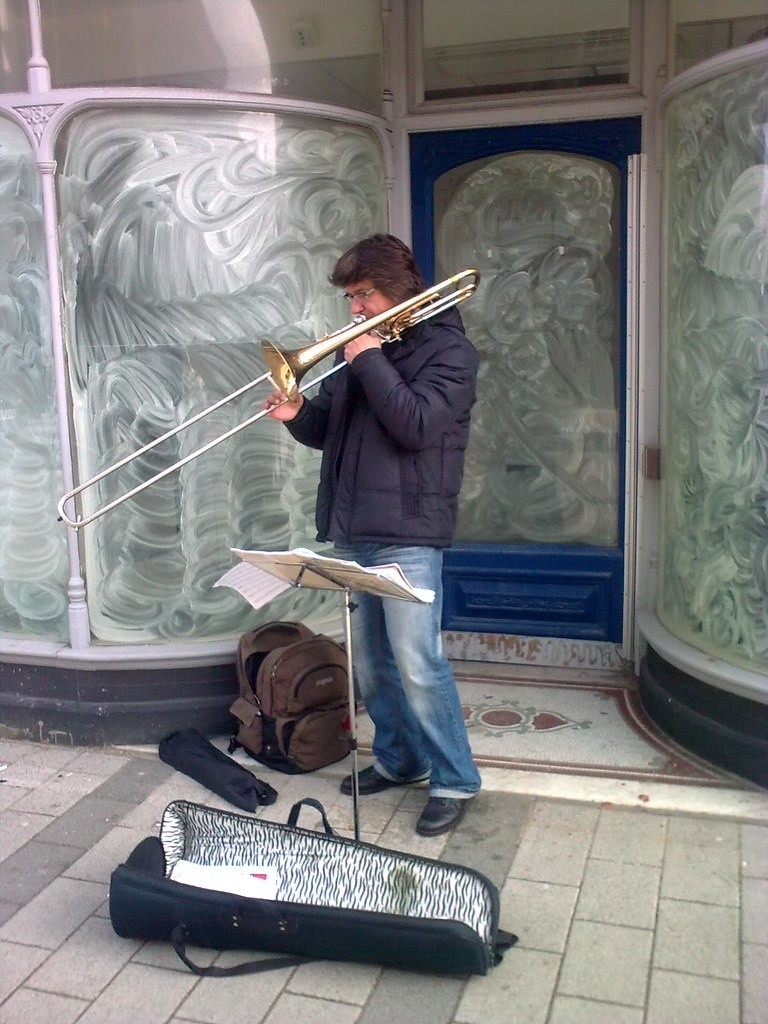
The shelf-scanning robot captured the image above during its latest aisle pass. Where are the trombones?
[56,266,485,528]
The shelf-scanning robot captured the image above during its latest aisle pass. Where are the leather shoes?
[340,764,430,796]
[416,796,478,837]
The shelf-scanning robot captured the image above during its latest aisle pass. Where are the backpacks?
[227,618,359,775]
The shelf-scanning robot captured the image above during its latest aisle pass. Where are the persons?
[264,232,481,835]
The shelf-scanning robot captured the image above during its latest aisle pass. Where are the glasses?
[343,287,377,303]
